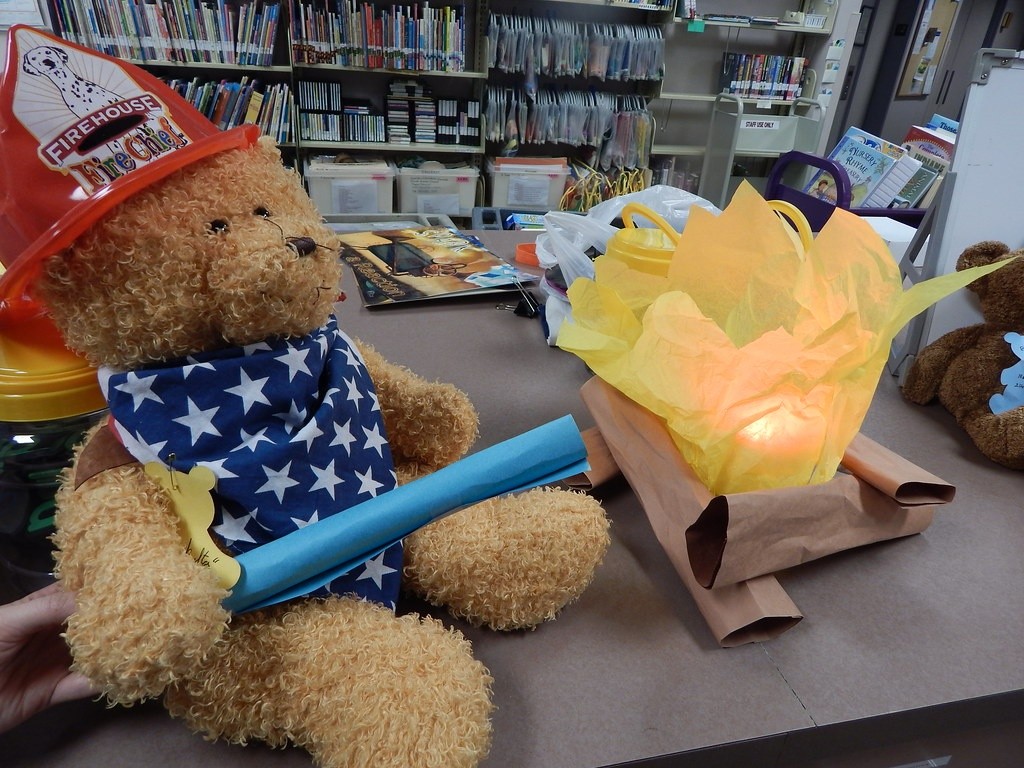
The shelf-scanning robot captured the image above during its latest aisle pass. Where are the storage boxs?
[484,151,572,213]
[302,156,395,215]
[391,158,479,218]
[766,149,937,232]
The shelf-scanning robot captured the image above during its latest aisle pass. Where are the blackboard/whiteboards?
[904,47,1024,356]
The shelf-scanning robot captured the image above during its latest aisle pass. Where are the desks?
[0,226,1023,768]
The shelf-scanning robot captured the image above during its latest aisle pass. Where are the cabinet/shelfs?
[33,0,836,221]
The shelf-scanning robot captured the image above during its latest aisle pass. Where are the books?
[651,155,701,198]
[608,0,778,23]
[289,0,467,72]
[54,0,285,67]
[155,74,297,146]
[717,47,811,103]
[800,113,962,213]
[297,74,483,150]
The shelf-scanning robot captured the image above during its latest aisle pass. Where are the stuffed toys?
[902,240,1024,470]
[0,21,615,767]
